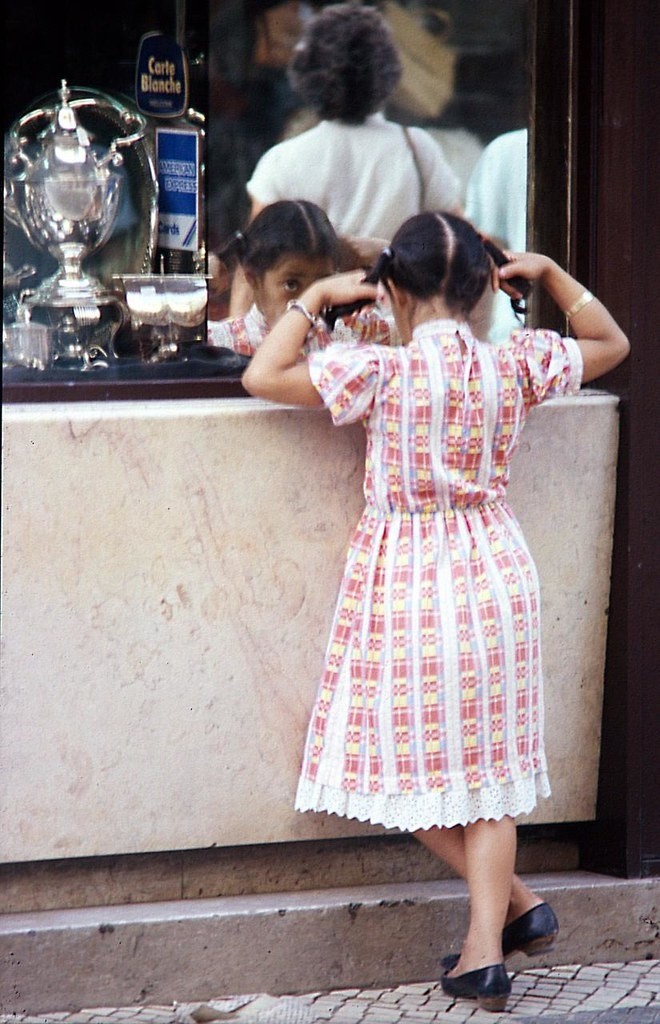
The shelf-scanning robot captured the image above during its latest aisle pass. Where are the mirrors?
[0,0,578,402]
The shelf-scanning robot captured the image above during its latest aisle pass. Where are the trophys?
[0,79,210,370]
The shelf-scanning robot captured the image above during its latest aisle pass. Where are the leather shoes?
[503,902,559,962]
[439,954,512,1012]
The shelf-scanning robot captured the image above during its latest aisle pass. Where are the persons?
[208,200,405,364]
[242,210,629,1013]
[463,128,526,332]
[229,4,463,322]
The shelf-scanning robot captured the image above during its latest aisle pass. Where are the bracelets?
[566,291,594,320]
[287,301,323,343]
